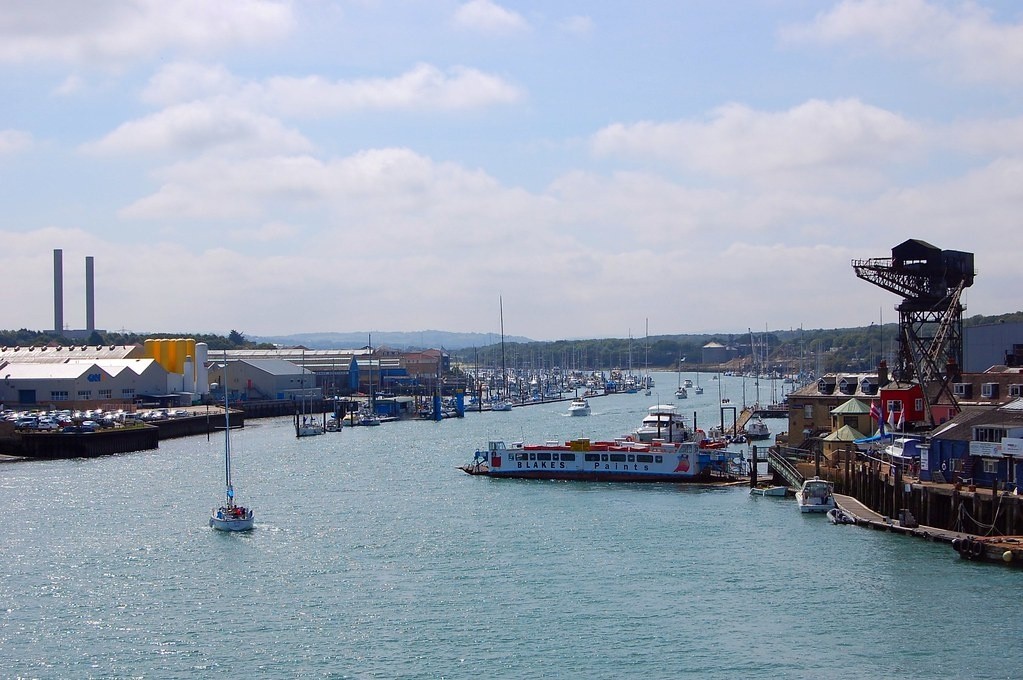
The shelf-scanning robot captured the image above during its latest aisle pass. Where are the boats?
[636,393,692,443]
[748,485,789,499]
[454,395,754,483]
[826,508,856,526]
[795,475,836,514]
[674,322,823,451]
[291,292,656,437]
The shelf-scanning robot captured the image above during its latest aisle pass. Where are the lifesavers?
[972,541,983,557]
[961,539,972,552]
[953,539,962,551]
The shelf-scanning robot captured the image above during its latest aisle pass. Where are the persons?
[227,504,240,519]
[910,455,917,479]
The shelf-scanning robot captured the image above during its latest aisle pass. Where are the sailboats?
[209,350,253,532]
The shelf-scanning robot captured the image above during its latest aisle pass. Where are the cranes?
[850,237,975,433]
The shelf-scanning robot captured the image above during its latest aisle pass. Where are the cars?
[0,401,192,432]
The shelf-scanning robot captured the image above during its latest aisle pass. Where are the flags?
[877,407,885,439]
[897,408,905,429]
[887,410,895,430]
[870,403,880,420]
